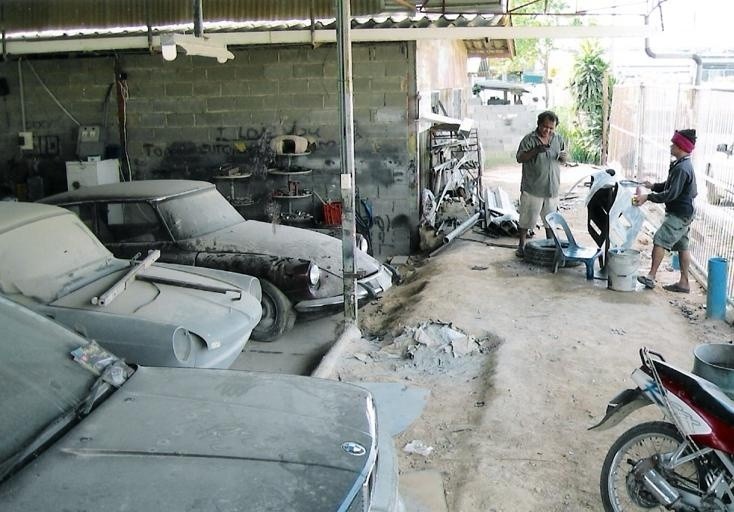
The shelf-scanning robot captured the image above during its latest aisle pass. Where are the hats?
[671,129,696,154]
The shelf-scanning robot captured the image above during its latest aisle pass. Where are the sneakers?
[636,275,656,288]
[662,282,690,293]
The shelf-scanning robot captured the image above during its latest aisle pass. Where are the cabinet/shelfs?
[211,149,319,228]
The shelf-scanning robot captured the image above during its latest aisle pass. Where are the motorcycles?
[591,344,732,511]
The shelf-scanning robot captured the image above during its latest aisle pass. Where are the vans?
[684,79,734,206]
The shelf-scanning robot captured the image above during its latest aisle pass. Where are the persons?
[632,127,700,293]
[513,109,570,259]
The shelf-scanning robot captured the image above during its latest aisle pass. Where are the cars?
[0,298,407,510]
[39,180,394,341]
[2,199,263,369]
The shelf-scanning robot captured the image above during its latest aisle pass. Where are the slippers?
[515,248,524,257]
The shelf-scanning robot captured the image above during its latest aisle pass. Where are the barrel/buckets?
[324,201,341,225]
[692,343,733,406]
[607,248,642,292]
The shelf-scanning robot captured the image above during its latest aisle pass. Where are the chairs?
[542,209,606,282]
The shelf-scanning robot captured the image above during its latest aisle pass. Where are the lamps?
[155,0,237,70]
